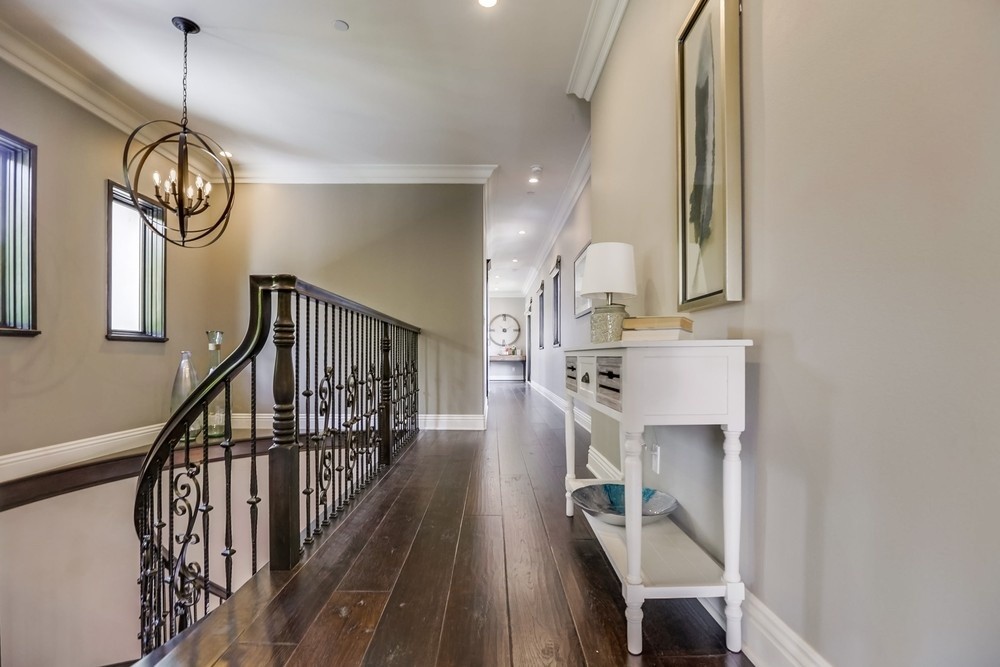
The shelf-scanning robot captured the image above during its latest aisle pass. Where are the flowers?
[505,345,517,352]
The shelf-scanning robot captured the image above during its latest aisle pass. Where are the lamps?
[122,16,235,249]
[579,242,638,341]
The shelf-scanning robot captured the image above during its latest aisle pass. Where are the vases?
[172,351,204,441]
[200,330,233,438]
[509,350,514,354]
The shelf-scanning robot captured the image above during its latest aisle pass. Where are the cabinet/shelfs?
[565,340,754,655]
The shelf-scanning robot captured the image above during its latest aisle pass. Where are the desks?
[490,355,526,361]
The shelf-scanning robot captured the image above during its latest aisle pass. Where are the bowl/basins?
[571,484,678,526]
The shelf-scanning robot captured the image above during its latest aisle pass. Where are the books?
[620,316,694,341]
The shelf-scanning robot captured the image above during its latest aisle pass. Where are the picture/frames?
[675,0,744,311]
[572,238,595,318]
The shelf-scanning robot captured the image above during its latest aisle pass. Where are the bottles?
[202,331,232,437]
[170,351,202,440]
